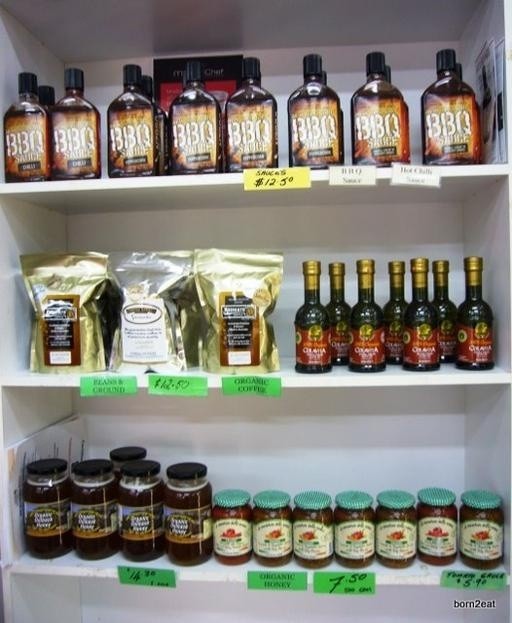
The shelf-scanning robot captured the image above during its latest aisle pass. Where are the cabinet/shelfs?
[2,1,512,623]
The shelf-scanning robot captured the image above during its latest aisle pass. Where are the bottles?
[287,53,344,165]
[4,71,55,182]
[166,61,223,175]
[420,48,483,166]
[47,68,101,179]
[107,64,167,176]
[222,57,279,175]
[349,53,410,165]
[293,255,495,375]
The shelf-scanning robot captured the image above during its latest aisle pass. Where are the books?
[152,54,244,175]
